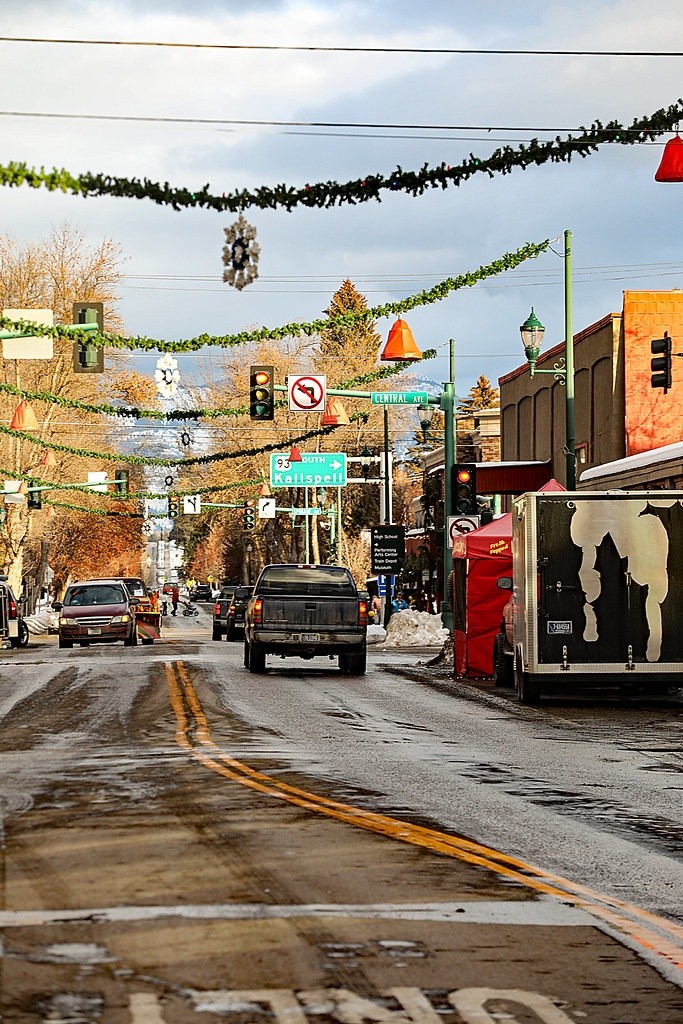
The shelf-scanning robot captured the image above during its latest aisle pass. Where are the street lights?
[415,335,457,463]
[318,487,337,566]
[246,544,253,587]
[519,229,578,494]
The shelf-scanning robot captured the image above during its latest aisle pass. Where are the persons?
[406,594,418,611]
[188,602,192,607]
[391,590,408,613]
[161,591,170,616]
[372,594,382,625]
[171,588,181,616]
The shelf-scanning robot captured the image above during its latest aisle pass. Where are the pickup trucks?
[245,563,368,675]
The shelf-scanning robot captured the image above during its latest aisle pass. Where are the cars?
[51,576,165,649]
[187,585,211,601]
[162,582,180,595]
[0,575,30,648]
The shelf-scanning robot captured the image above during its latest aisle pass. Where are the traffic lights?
[168,494,179,519]
[242,496,257,529]
[249,366,275,419]
[451,463,479,516]
[650,330,673,390]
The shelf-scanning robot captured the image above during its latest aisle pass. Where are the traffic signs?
[268,452,348,487]
[259,498,276,519]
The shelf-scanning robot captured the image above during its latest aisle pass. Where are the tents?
[452,478,571,679]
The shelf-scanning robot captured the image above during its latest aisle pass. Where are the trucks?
[492,489,683,706]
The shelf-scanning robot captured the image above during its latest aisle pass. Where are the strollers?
[178,600,198,616]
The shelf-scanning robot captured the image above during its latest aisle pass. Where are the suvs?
[212,586,254,641]
